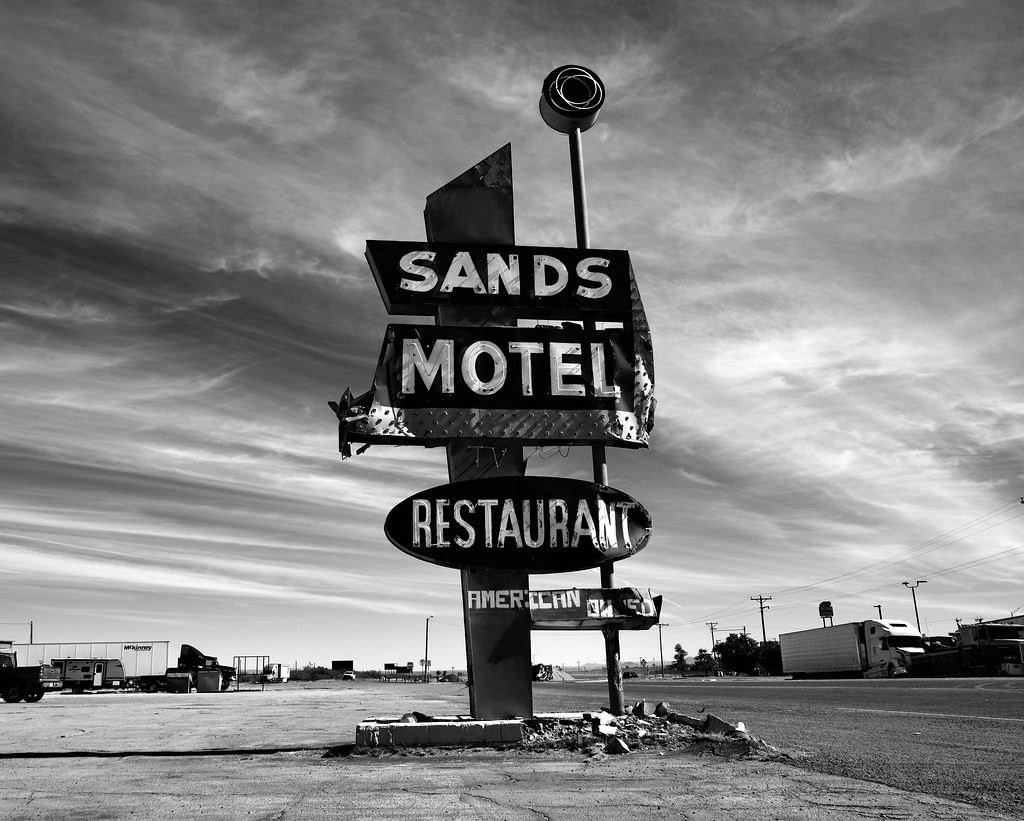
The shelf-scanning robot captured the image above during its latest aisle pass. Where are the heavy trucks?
[779,618,929,680]
[11,641,236,693]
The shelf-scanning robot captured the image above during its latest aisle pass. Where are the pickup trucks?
[0,652,64,703]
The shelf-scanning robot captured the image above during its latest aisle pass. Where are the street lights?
[424,616,434,685]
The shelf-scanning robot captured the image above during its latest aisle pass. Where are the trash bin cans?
[715,670,724,677]
[166,673,193,693]
[197,671,223,692]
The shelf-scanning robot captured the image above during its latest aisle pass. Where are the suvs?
[623,671,639,678]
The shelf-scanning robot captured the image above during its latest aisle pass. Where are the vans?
[343,671,356,680]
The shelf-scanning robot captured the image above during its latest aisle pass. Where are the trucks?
[262,664,290,683]
[39,657,126,694]
